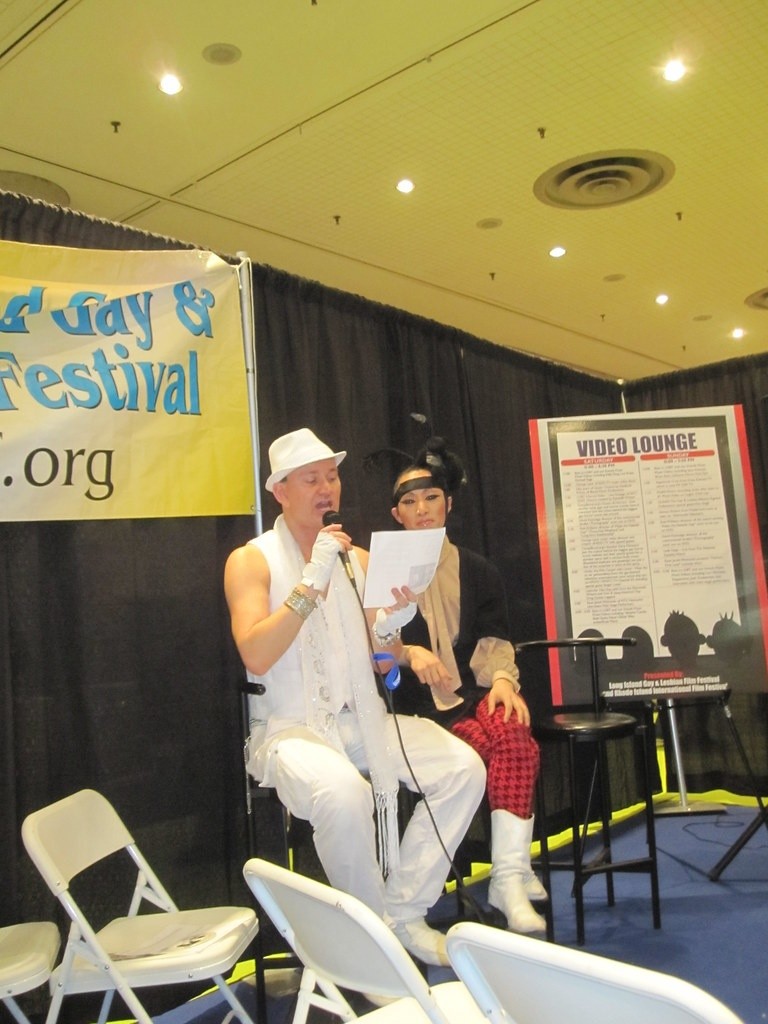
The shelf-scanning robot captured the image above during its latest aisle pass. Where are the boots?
[488,808,549,933]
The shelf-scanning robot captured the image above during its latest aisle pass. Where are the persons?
[375,436,548,934]
[223,428,487,1006]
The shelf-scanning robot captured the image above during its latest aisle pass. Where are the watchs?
[372,625,401,647]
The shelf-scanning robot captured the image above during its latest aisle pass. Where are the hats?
[264,427,347,491]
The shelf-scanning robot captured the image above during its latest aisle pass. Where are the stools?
[240,677,491,1024]
[510,635,662,948]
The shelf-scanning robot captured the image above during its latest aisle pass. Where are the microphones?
[323,510,356,586]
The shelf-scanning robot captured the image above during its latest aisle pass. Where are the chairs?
[20,788,259,1024]
[0,921,61,1024]
[445,921,745,1024]
[243,858,488,1024]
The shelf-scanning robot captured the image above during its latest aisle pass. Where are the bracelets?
[284,588,318,621]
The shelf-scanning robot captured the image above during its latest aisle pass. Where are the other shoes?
[384,921,450,966]
